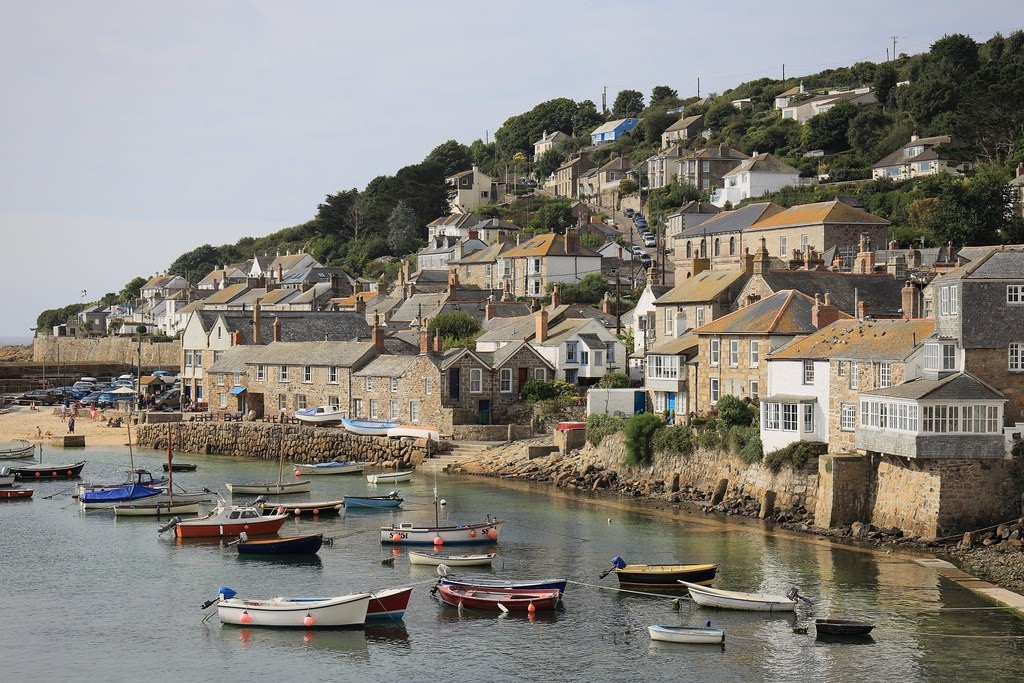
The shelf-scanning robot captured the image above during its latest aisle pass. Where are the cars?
[13,369,188,410]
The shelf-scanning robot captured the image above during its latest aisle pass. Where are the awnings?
[229,387,247,394]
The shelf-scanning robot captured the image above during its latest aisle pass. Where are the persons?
[67,416,75,434]
[71,406,76,419]
[73,402,80,417]
[64,397,70,407]
[108,418,114,426]
[61,403,68,423]
[138,392,156,411]
[37,426,41,436]
[89,403,97,422]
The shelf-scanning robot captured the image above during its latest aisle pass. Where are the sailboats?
[676,579,814,611]
[225,423,312,495]
[70,416,218,516]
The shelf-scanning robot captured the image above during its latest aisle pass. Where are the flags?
[82,290,87,297]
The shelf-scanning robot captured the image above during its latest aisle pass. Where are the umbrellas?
[111,386,139,395]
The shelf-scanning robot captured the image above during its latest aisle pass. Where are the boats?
[814,617,876,633]
[201,592,372,628]
[647,621,726,642]
[340,418,404,438]
[0,468,17,486]
[293,461,366,475]
[343,490,405,507]
[250,494,347,520]
[0,488,35,500]
[366,470,413,483]
[293,405,345,424]
[430,584,560,610]
[0,444,36,459]
[155,504,290,538]
[436,563,569,600]
[226,530,324,555]
[387,425,440,443]
[6,442,86,478]
[379,486,506,546]
[556,420,587,431]
[409,551,497,566]
[218,585,414,620]
[598,556,721,589]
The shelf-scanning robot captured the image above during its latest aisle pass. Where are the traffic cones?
[32,400,37,411]
